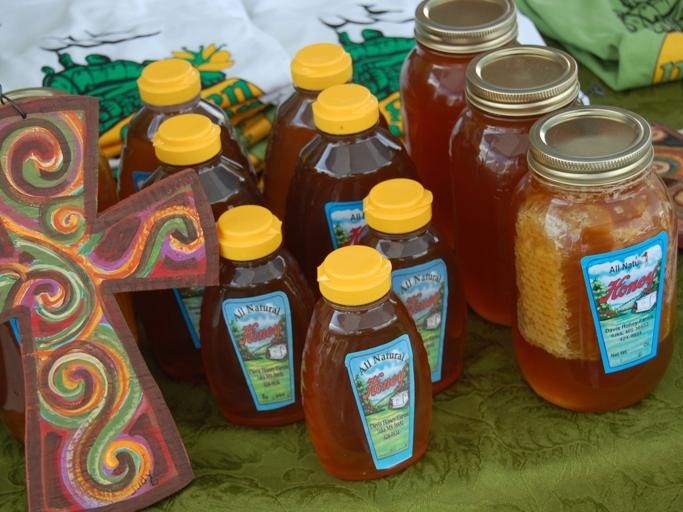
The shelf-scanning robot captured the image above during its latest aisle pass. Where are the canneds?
[399,1,523,253]
[509,104,678,413]
[446,46,590,328]
